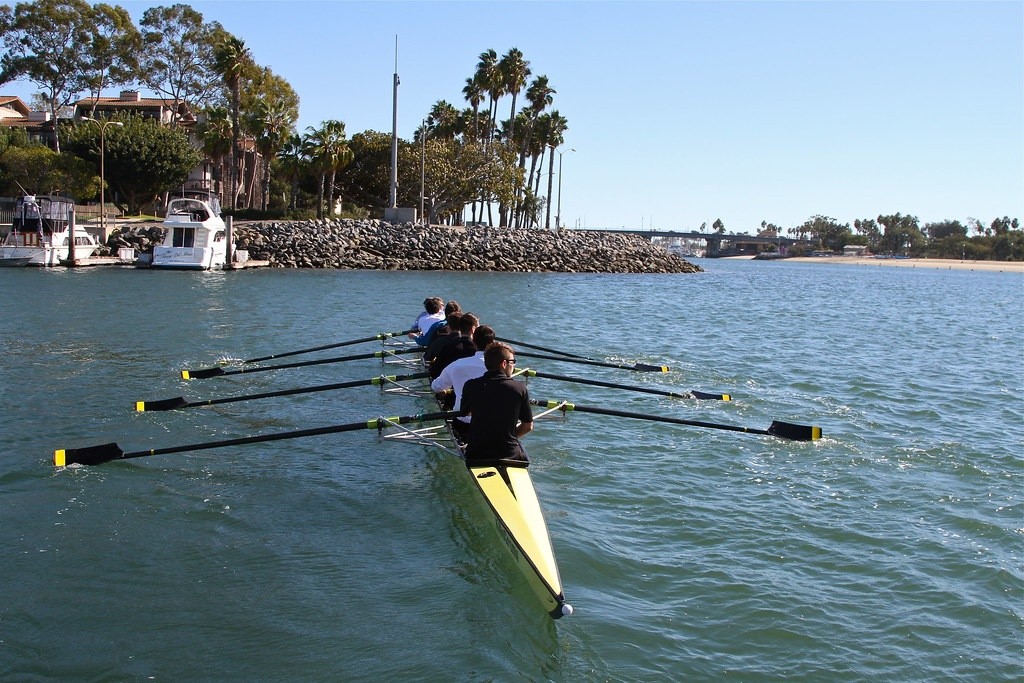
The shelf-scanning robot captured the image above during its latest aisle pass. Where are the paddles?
[513,349,668,373]
[494,336,594,359]
[532,396,824,441]
[243,329,415,362]
[181,345,424,379]
[516,367,732,401]
[134,371,428,413]
[53,410,468,468]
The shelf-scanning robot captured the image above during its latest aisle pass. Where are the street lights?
[546,143,576,228]
[80,115,124,228]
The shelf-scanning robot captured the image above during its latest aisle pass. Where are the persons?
[461,343,533,462]
[430,325,495,443]
[409,298,480,403]
[200,196,211,209]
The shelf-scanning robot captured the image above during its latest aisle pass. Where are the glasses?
[497,358,517,366]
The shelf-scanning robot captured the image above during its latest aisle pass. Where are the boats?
[150,197,239,271]
[422,351,573,621]
[0,180,100,267]
